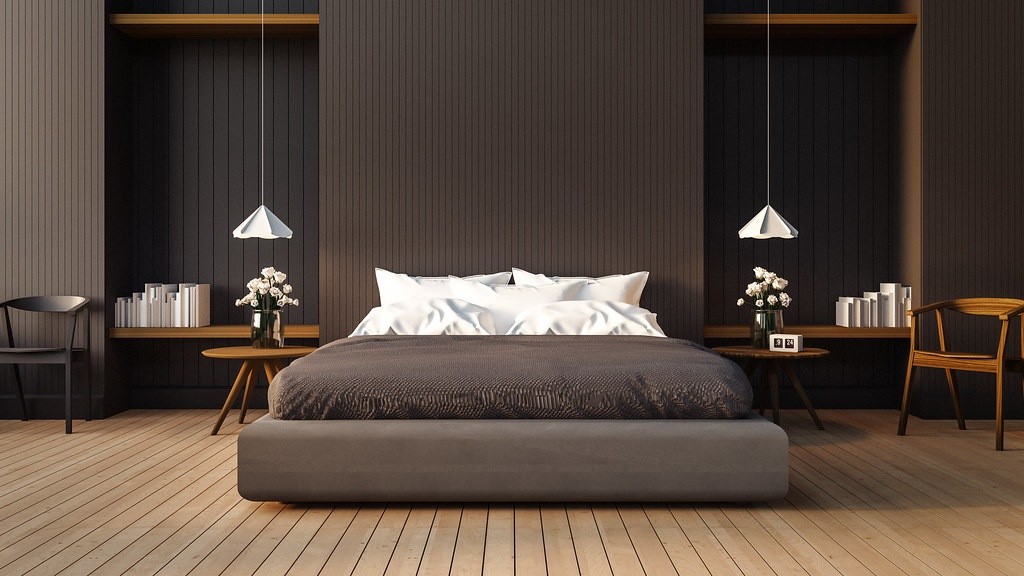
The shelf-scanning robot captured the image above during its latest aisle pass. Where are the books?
[115,283,210,327]
[836,283,912,327]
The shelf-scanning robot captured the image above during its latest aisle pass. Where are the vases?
[749,310,783,349]
[251,309,285,348]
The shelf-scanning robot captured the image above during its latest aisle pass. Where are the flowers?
[235,267,299,344]
[736,267,792,345]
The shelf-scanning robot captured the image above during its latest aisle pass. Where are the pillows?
[347,267,669,339]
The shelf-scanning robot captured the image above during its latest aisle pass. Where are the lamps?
[233,0,293,240]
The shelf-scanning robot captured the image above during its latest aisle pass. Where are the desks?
[711,346,830,431]
[202,346,319,435]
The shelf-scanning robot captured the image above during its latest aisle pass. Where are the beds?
[238,336,791,504]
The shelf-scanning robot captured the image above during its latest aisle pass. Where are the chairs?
[0,295,91,434]
[898,298,1024,452]
